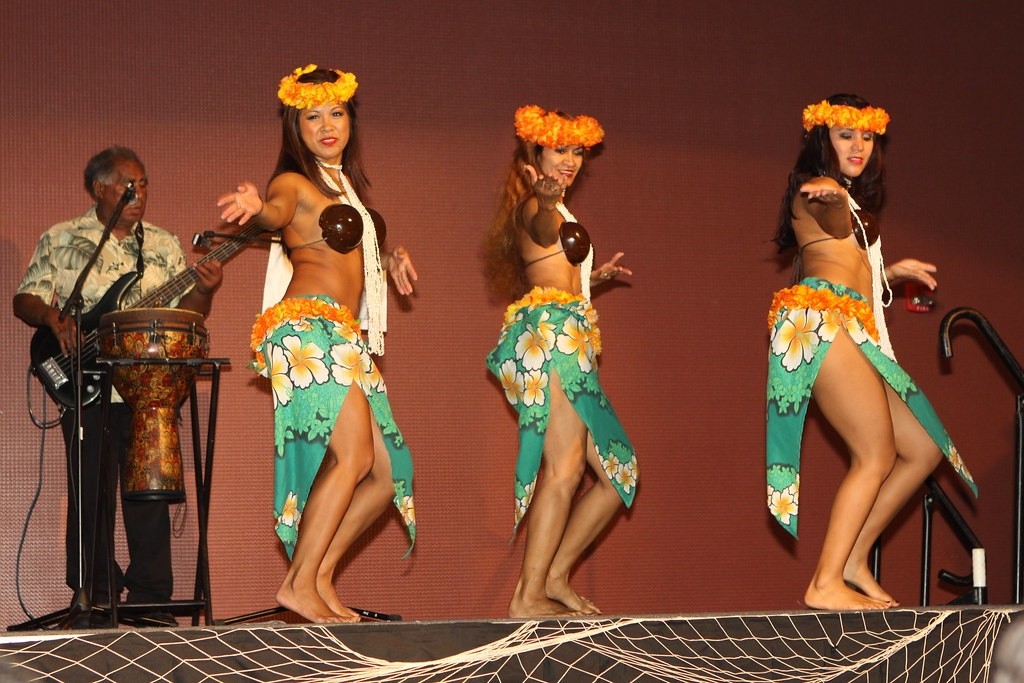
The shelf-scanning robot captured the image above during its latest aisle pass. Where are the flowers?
[274,63,359,108]
[801,99,891,136]
[502,285,603,355]
[249,297,364,374]
[766,285,880,343]
[513,105,605,149]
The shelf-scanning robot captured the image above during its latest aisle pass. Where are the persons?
[486,105,639,616]
[766,94,978,609]
[217,63,418,623]
[13,146,222,623]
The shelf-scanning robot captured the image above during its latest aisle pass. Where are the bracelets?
[252,195,264,217]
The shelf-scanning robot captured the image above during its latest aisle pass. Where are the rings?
[551,186,555,192]
[237,202,241,208]
[603,272,608,277]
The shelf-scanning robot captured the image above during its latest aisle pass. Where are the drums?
[100,306,211,507]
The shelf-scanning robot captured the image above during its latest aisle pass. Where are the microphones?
[128,180,138,205]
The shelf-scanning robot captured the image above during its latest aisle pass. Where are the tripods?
[6,193,178,631]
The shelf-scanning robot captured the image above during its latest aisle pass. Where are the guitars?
[29,221,268,414]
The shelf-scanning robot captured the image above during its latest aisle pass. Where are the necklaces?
[556,187,594,303]
[316,161,384,356]
[844,178,897,363]
